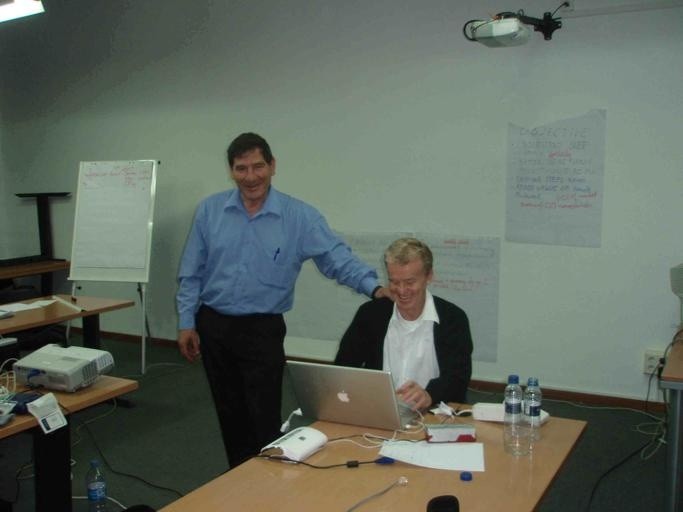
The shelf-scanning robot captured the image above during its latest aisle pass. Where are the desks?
[0,191,72,345]
[154,402,589,512]
[0,364,139,512]
[0,293,135,411]
[658,330,681,502]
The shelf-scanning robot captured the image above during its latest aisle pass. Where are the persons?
[333,238,474,413]
[175,131,394,472]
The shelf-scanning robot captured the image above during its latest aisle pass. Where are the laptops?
[286,359,425,434]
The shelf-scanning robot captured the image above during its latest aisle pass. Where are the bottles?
[85,460,106,512]
[504,375,523,430]
[522,377,541,430]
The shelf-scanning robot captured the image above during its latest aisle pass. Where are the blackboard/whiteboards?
[67,158,156,283]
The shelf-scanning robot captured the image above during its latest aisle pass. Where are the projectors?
[12,343,115,395]
[460,19,532,50]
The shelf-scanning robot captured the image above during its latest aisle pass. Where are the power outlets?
[643,350,668,377]
